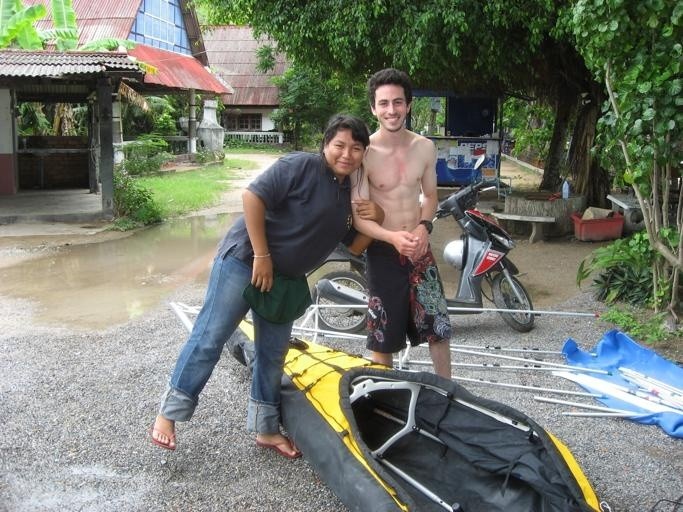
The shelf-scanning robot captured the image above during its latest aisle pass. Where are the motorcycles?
[305,151,535,332]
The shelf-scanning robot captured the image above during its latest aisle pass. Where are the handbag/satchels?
[243,269,313,324]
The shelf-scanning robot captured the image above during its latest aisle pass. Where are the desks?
[16,145,91,188]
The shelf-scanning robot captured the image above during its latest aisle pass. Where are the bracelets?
[251,253,271,259]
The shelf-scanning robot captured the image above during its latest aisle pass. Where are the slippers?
[256,432,303,459]
[150,419,176,450]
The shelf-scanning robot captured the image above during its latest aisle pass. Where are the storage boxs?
[572,211,622,242]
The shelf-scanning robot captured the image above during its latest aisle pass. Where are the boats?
[226,321,613,512]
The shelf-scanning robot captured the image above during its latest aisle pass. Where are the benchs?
[490,210,555,244]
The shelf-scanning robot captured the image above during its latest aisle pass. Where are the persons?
[345,66,454,380]
[149,114,386,458]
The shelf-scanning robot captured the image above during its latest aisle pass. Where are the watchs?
[418,219,434,234]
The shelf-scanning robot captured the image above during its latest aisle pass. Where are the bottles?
[562,180,569,199]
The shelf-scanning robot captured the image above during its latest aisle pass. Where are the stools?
[606,193,645,234]
